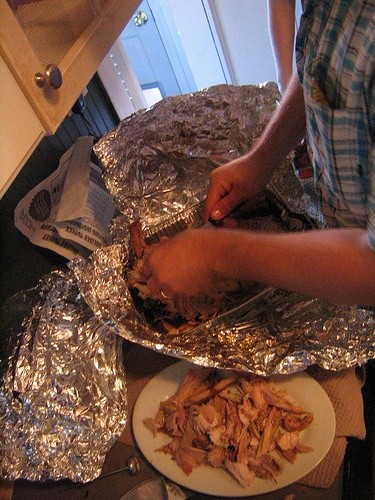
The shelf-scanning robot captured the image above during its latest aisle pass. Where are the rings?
[159,290,176,301]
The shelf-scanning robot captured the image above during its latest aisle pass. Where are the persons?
[267,0,306,153]
[139,0,375,314]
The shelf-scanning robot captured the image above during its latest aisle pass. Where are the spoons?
[58,456,140,493]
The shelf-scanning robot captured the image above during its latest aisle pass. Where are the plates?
[132,359,337,498]
